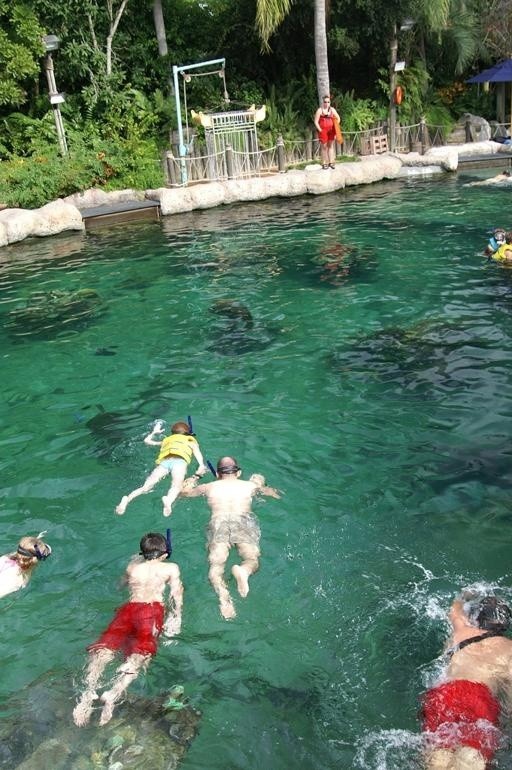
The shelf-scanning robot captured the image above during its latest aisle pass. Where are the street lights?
[38,33,70,158]
[387,15,419,151]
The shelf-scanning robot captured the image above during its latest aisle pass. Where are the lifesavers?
[395,86,402,104]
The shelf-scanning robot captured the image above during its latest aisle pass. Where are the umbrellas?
[463,58,511,85]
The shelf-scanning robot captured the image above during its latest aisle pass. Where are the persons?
[488,232,512,262]
[0,529,54,599]
[423,579,512,769]
[71,529,183,726]
[183,454,283,621]
[463,169,511,188]
[314,96,341,170]
[116,413,207,517]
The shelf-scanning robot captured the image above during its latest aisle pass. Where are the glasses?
[17,543,53,560]
[324,101,330,103]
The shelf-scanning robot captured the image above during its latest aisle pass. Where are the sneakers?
[323,164,327,169]
[329,163,335,169]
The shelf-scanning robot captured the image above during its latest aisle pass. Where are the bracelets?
[195,472,203,479]
[262,483,268,488]
